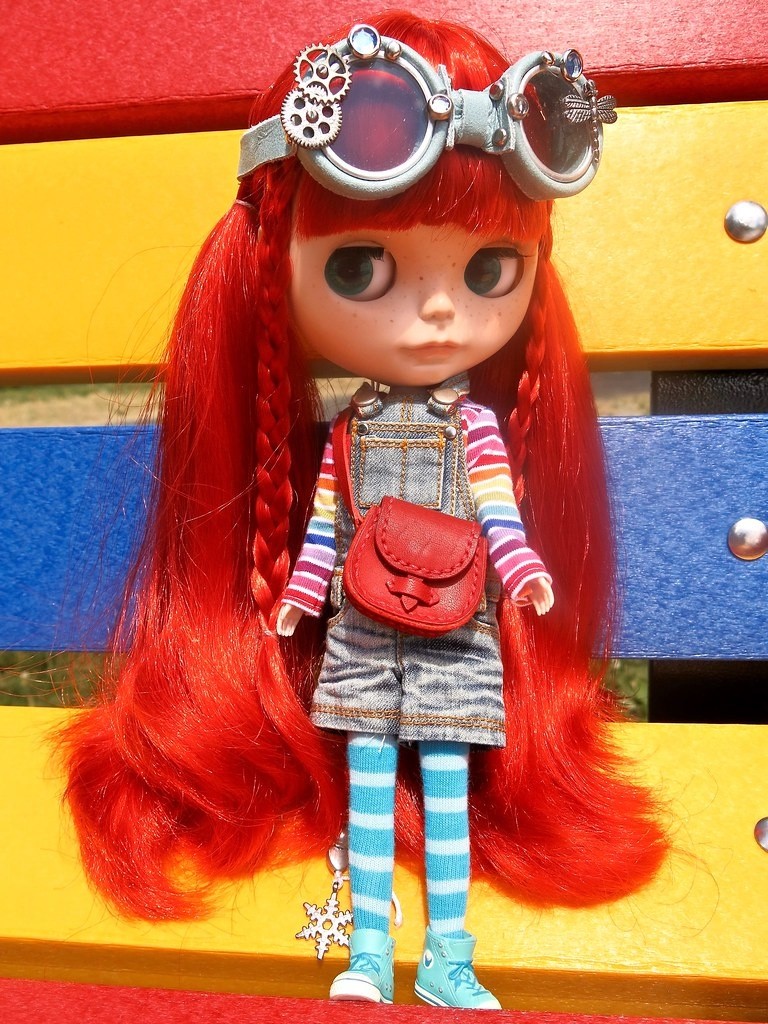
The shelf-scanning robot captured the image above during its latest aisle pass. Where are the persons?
[66,11,668,1010]
